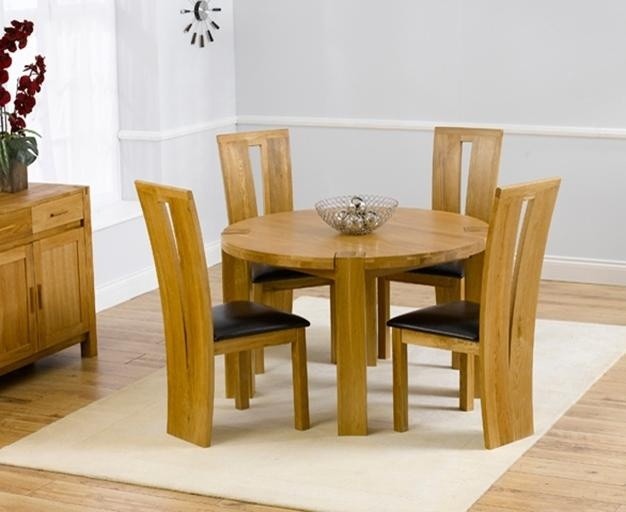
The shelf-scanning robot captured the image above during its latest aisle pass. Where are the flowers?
[0,19,47,178]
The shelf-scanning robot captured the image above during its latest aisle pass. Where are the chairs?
[135,178,310,449]
[216,128,337,374]
[375,127,502,360]
[385,177,560,449]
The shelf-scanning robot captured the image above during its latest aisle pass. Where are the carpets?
[1,296,625,512]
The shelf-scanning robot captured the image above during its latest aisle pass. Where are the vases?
[1,161,27,194]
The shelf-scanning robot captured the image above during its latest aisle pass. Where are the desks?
[219,207,490,436]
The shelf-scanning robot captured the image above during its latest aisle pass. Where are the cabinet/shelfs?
[0,182,97,374]
